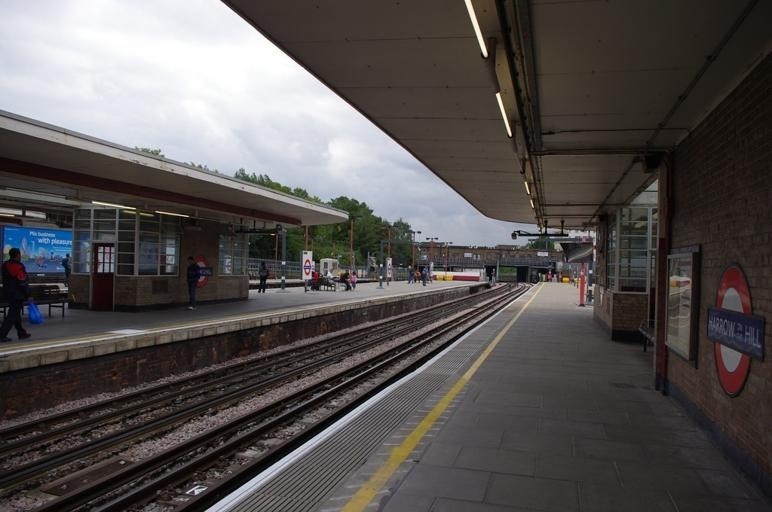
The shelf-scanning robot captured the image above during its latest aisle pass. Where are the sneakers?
[0,337,11,342]
[19,334,31,339]
[187,306,193,310]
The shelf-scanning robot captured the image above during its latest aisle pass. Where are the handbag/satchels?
[196,272,208,288]
[28,303,42,324]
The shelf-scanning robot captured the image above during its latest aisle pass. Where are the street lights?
[349,215,362,264]
[426,237,438,262]
[385,225,400,258]
[442,242,452,271]
[407,230,421,266]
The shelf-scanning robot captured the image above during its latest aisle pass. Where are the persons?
[257,262,270,293]
[491,268,497,286]
[309,271,323,291]
[349,270,358,288]
[339,272,352,291]
[1,247,34,342]
[408,264,434,285]
[60,254,71,287]
[186,255,201,310]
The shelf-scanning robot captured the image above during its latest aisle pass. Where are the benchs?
[0,284,73,320]
